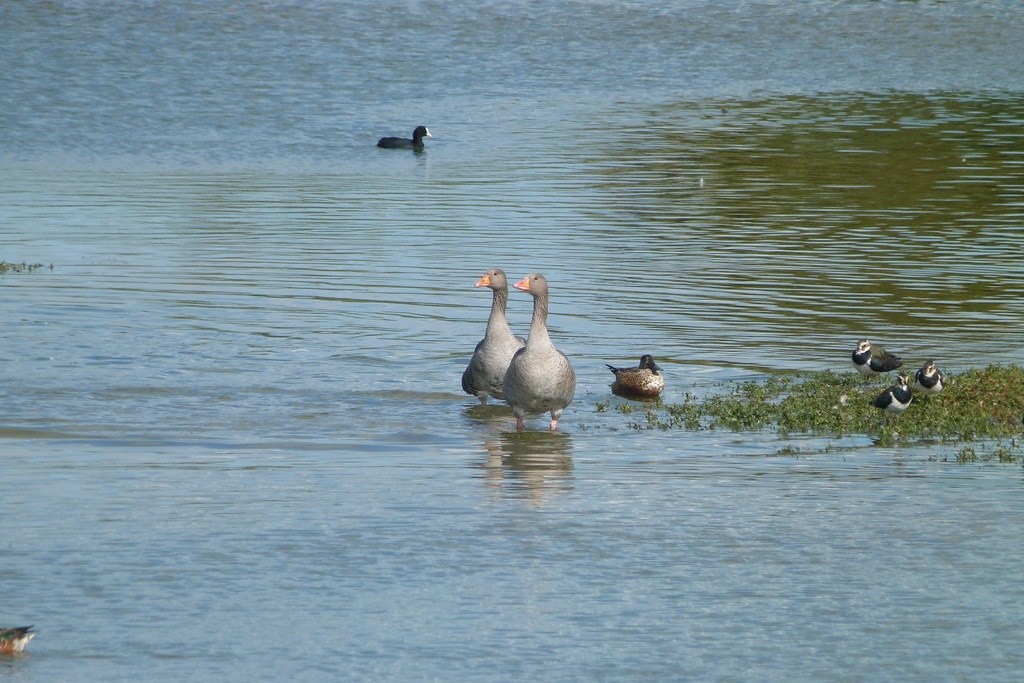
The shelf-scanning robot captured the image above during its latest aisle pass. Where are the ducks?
[605,354,665,397]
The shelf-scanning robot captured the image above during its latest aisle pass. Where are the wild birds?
[869,372,913,427]
[461,268,527,408]
[913,357,946,401]
[851,338,904,385]
[375,126,433,149]
[502,272,577,431]
[0,624,41,655]
